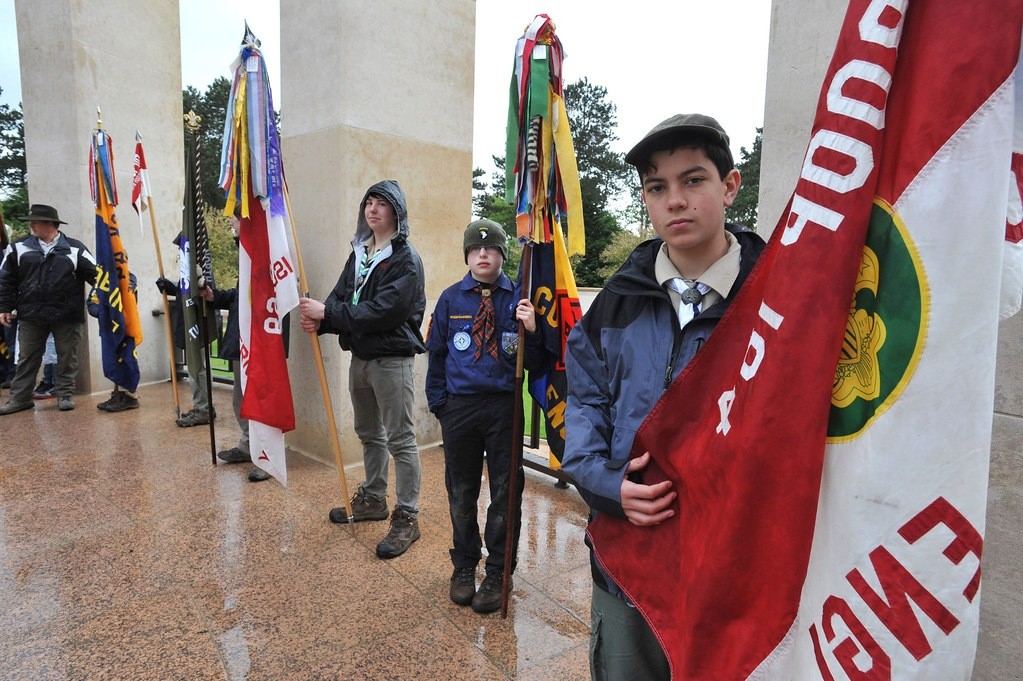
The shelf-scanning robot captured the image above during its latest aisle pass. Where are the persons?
[300,179,428,561]
[424,218,536,617]
[563,115,773,681]
[0,204,97,418]
[201,217,292,483]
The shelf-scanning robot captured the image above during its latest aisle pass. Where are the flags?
[182,47,300,494]
[86,129,149,396]
[585,0,1023,681]
[502,11,588,487]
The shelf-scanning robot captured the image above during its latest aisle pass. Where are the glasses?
[466,246,501,256]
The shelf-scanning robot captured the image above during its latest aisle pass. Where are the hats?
[625,113,730,166]
[463,219,509,263]
[18,204,68,224]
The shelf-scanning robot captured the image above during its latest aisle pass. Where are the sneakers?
[218,447,251,462]
[97,390,125,409]
[175,409,210,427]
[472,571,513,613]
[450,567,476,606]
[33,381,57,398]
[248,467,272,481]
[106,394,140,411]
[0,399,35,415]
[58,396,75,410]
[376,505,420,559]
[1,381,11,389]
[181,406,216,420]
[329,485,390,523]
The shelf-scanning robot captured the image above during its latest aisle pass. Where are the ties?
[354,248,382,305]
[667,276,714,331]
[472,284,501,364]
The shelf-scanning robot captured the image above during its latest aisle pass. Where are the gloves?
[156,277,178,296]
